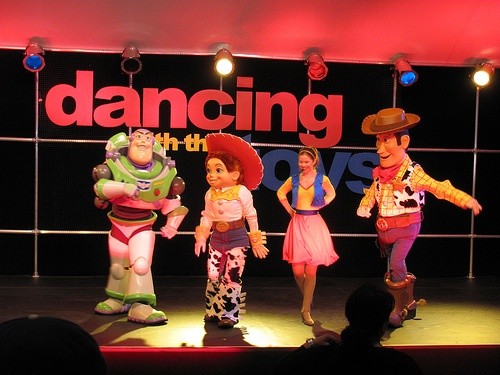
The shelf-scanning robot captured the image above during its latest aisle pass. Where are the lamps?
[396,59,419,88]
[306,53,329,82]
[22,45,46,72]
[469,61,497,88]
[213,49,237,78]
[120,47,143,75]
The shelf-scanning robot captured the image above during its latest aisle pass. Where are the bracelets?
[306,337,313,345]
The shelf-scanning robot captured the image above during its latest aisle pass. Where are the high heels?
[299,315,301,316]
[300,310,314,325]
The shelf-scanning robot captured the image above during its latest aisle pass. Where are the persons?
[274,284,423,375]
[92,129,188,323]
[276,146,340,326]
[194,133,270,329]
[356,108,482,326]
